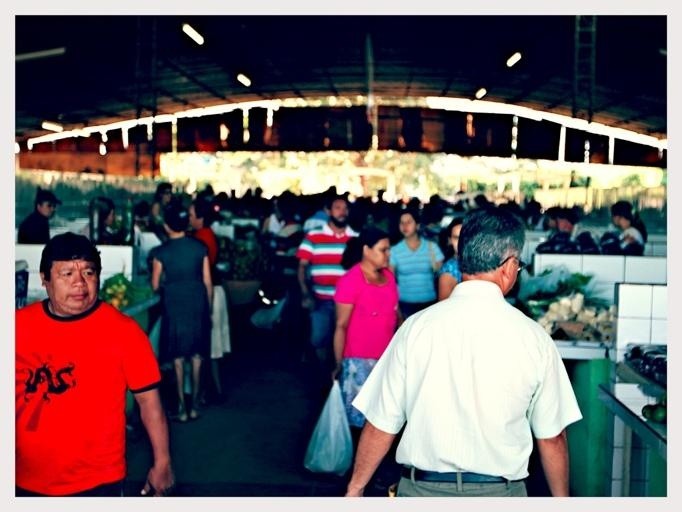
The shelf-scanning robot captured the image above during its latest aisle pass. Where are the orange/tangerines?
[642,405,667,422]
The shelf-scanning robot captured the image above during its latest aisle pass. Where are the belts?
[400,468,512,483]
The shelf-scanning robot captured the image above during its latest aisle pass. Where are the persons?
[344,208,582,498]
[332,226,398,484]
[241,184,648,379]
[16,231,176,497]
[16,181,235,423]
